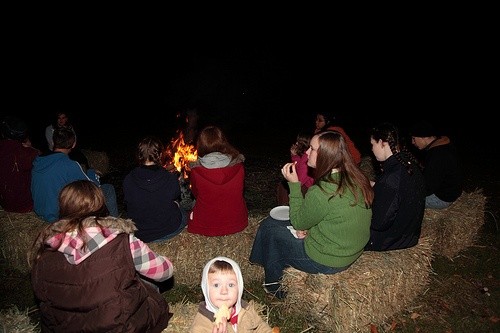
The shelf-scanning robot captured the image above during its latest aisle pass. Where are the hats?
[410,119,438,137]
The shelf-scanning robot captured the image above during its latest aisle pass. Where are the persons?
[409,125,466,209]
[122,138,188,243]
[190,257,279,333]
[276,110,361,206]
[248,129,375,300]
[30,127,118,223]
[29,180,174,333]
[364,130,426,252]
[186,125,248,237]
[0,132,45,213]
[45,112,88,154]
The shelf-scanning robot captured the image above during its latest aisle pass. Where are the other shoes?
[264,282,283,300]
